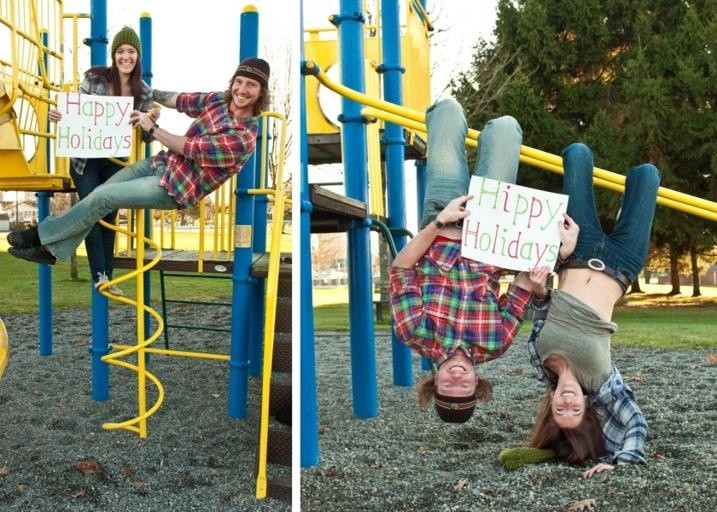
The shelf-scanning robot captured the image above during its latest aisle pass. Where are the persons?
[528,141,661,479]
[389,97,581,424]
[7,58,271,265]
[47,23,159,296]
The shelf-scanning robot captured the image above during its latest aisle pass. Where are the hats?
[434,392,476,423]
[499,447,556,470]
[112,26,141,56]
[233,57,270,88]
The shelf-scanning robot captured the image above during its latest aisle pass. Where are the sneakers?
[93,272,124,296]
[7,224,57,265]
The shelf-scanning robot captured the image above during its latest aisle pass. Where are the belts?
[420,214,464,230]
[559,258,630,290]
